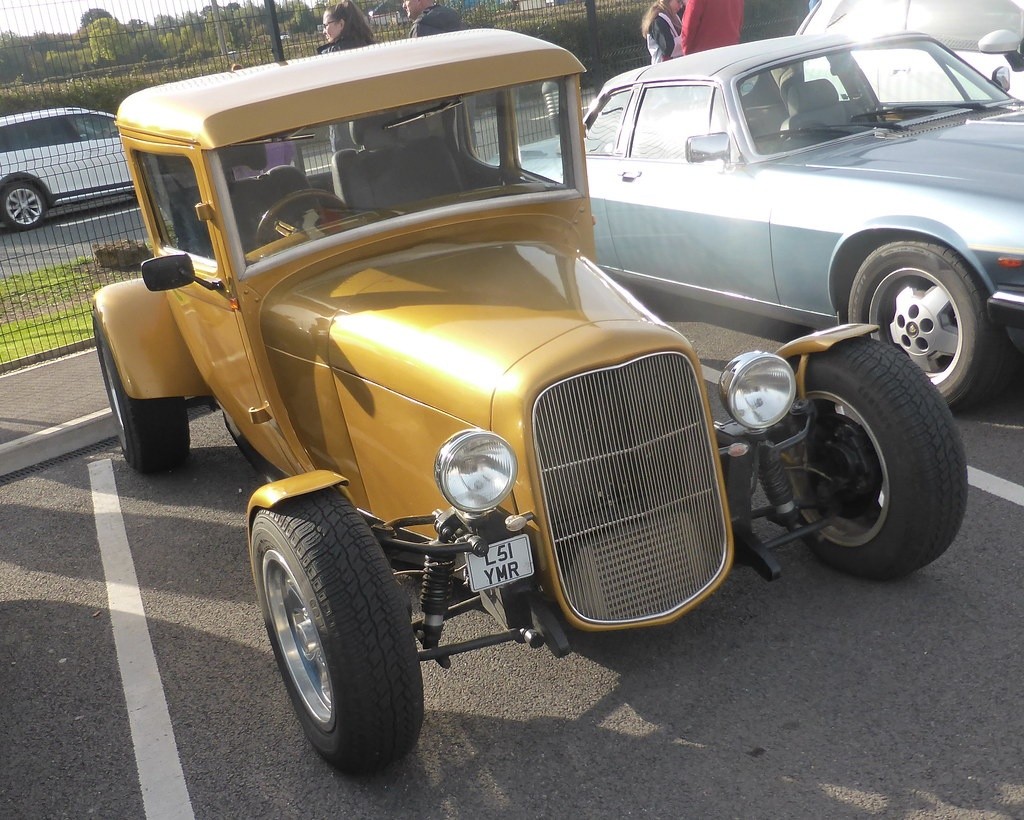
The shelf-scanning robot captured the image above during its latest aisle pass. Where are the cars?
[794,0,1024,108]
[0,106,136,232]
[90,27,969,780]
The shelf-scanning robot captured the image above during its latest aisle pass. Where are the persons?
[402,0,477,152]
[681,0,744,56]
[316,0,377,154]
[641,0,686,65]
[227,64,294,180]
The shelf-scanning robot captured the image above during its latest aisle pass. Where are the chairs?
[781,76,871,148]
[169,165,321,257]
[330,111,462,208]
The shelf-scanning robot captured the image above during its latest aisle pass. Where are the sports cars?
[487,29,1024,416]
